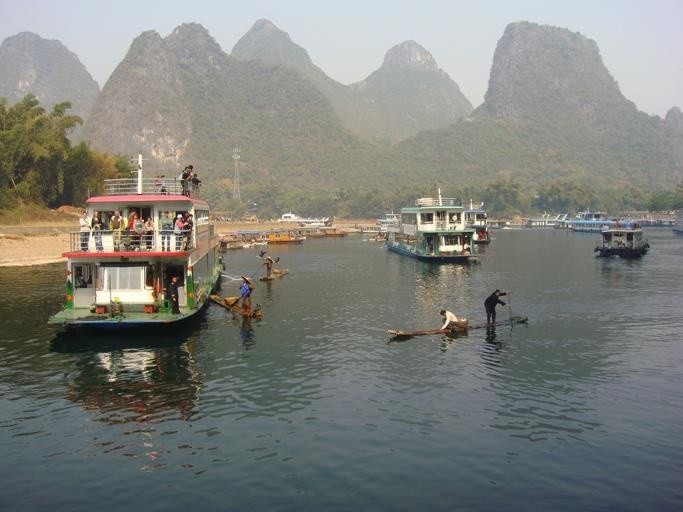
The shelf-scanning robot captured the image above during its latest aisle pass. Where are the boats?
[385,316,527,337]
[259,268,288,280]
[570,219,641,233]
[46,152,226,335]
[209,295,262,320]
[373,187,491,263]
[220,226,345,250]
[593,219,649,257]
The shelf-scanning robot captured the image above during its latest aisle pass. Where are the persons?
[161,175,167,194]
[263,256,273,277]
[241,319,253,350]
[239,275,251,314]
[260,250,265,258]
[487,326,502,351]
[167,277,183,314]
[440,310,459,332]
[178,164,202,198]
[79,274,92,288]
[484,289,510,324]
[439,333,458,352]
[464,242,470,252]
[79,207,194,253]
[145,270,174,288]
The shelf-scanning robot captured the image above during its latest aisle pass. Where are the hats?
[176,214,182,218]
[267,257,271,261]
[241,275,251,284]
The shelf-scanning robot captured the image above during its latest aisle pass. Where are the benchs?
[95,291,154,314]
